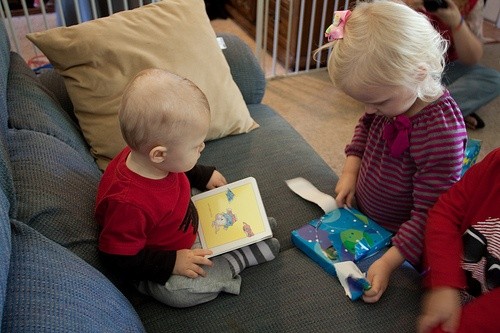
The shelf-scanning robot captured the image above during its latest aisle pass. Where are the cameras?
[423,0,448,12]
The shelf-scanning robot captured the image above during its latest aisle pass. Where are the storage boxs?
[291,204,393,275]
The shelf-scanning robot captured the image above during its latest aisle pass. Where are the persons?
[322,0,467,304]
[94,68,282,308]
[415,147,500,333]
[398,0,500,130]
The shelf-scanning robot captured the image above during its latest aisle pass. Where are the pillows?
[8,128,104,271]
[26,0,260,174]
[6,50,95,166]
[0,218,147,333]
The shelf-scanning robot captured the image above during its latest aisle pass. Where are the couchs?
[0,15,427,333]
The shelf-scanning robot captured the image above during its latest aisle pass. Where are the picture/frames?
[190,176,273,259]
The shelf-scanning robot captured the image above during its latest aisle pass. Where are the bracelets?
[448,17,464,30]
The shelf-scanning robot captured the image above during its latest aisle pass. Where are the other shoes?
[465,112,485,129]
